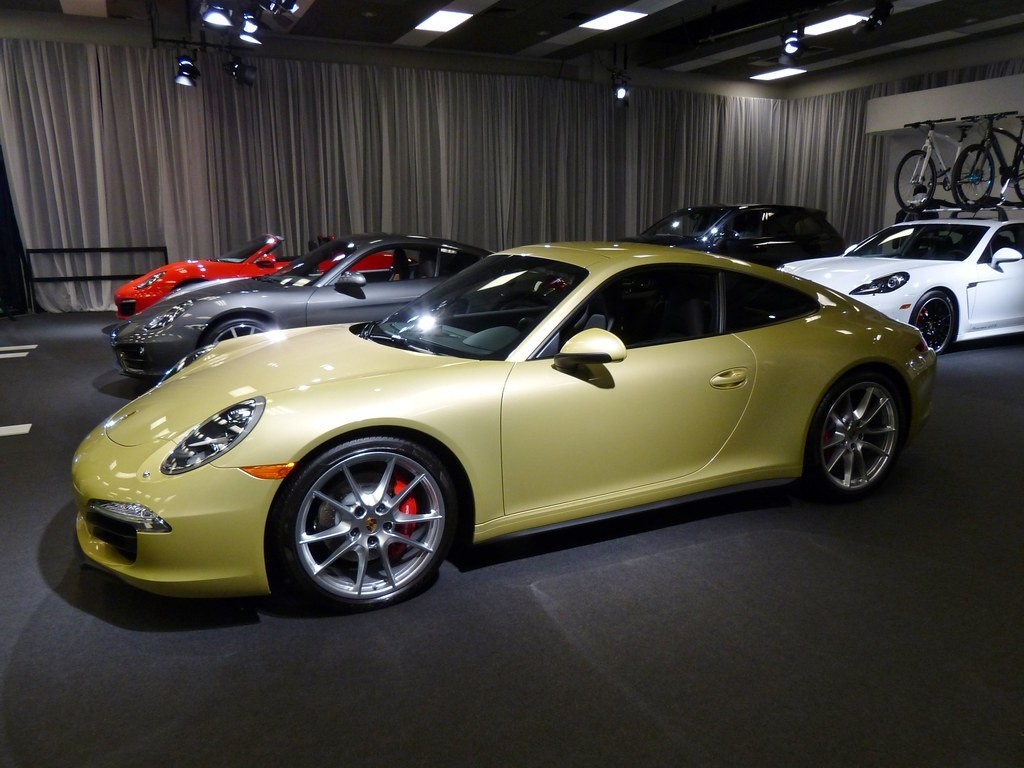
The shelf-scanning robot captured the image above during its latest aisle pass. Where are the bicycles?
[893,117,992,214]
[950,111,1024,213]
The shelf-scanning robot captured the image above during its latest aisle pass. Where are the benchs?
[643,262,777,338]
[654,278,706,339]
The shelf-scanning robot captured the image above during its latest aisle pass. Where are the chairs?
[389,247,405,280]
[584,282,634,343]
[921,234,952,259]
[307,239,319,252]
[735,214,765,240]
[418,246,434,278]
[992,236,1012,252]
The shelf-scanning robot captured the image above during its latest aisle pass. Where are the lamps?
[851,1,894,43]
[200,0,300,46]
[220,49,258,87]
[174,47,201,89]
[777,17,807,53]
[607,67,633,107]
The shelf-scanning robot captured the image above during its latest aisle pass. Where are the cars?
[611,203,848,270]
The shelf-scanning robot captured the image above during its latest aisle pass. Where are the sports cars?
[70,238,938,617]
[111,231,421,324]
[109,233,571,381]
[774,206,1024,357]
